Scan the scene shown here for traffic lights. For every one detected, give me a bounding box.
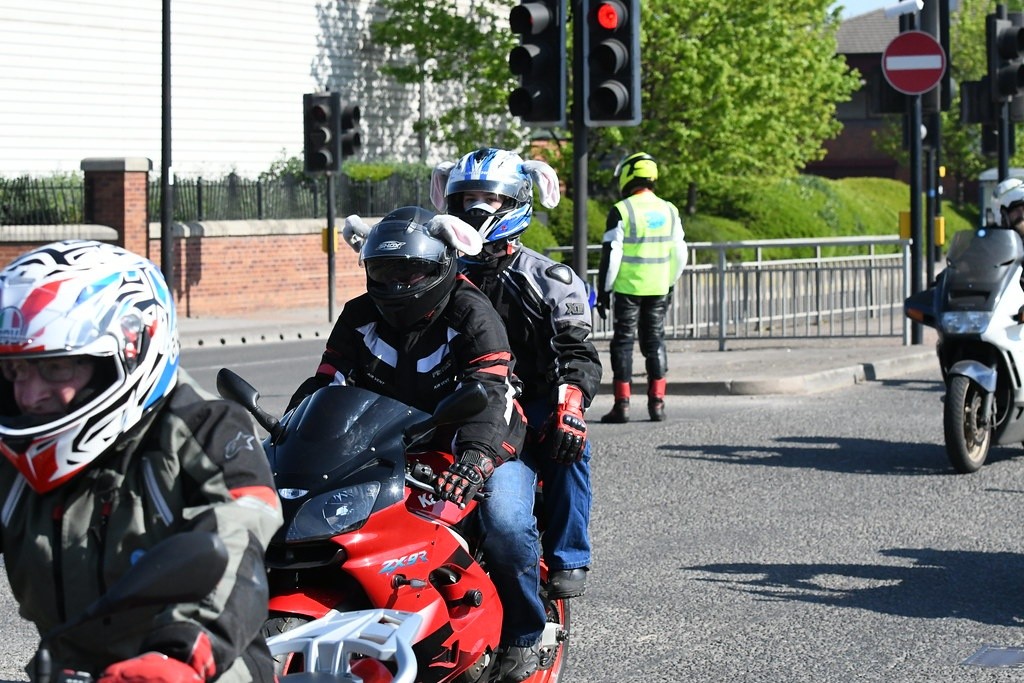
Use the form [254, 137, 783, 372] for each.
[507, 0, 568, 126]
[987, 14, 1024, 100]
[302, 91, 341, 172]
[940, 0, 960, 112]
[586, 1, 643, 129]
[341, 104, 363, 164]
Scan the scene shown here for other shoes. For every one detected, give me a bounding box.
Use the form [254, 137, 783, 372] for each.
[601, 403, 629, 423]
[548, 567, 586, 599]
[648, 399, 666, 420]
[499, 641, 540, 682]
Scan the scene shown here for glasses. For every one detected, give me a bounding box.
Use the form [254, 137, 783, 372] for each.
[0, 358, 104, 383]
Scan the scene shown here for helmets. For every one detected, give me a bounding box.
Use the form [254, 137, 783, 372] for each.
[444, 149, 533, 245]
[359, 206, 457, 329]
[613, 152, 658, 199]
[0, 239, 181, 499]
[990, 178, 1024, 227]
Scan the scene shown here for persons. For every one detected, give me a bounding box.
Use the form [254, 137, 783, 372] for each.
[0, 239, 307, 683]
[311, 207, 546, 682]
[936, 178, 1024, 292]
[596, 151, 688, 423]
[430, 148, 602, 599]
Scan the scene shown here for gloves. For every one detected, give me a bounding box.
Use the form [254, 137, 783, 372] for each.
[430, 449, 504, 510]
[668, 287, 674, 303]
[597, 291, 610, 319]
[536, 382, 590, 466]
[98, 622, 216, 683]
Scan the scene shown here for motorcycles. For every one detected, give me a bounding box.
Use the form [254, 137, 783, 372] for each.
[903, 222, 1024, 472]
[0, 366, 571, 683]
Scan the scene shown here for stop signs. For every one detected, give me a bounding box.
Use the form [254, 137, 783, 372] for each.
[883, 30, 945, 96]
